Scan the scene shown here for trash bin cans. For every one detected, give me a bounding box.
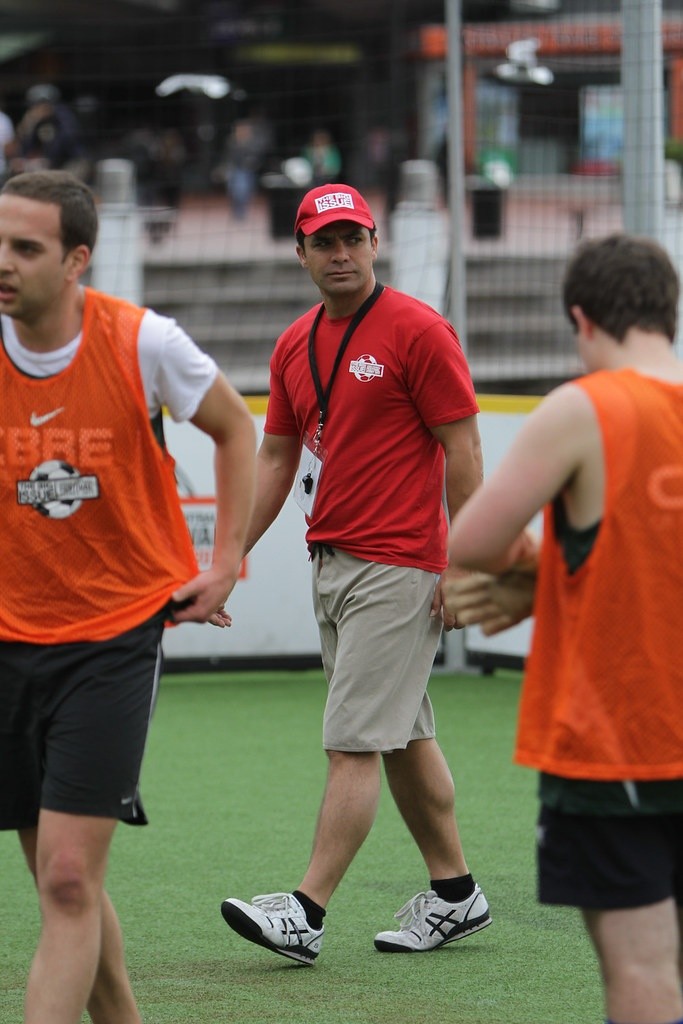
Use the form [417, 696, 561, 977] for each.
[471, 186, 504, 237]
[261, 187, 306, 242]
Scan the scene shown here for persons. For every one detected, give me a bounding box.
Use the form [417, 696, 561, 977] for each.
[0, 79, 399, 233]
[208, 184, 494, 965]
[441, 228, 683, 1023]
[0, 167, 259, 1024]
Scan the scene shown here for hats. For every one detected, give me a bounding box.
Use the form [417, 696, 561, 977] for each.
[294, 184, 374, 236]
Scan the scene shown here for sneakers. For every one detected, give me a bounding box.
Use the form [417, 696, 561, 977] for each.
[221, 892, 324, 966]
[373, 883, 492, 952]
